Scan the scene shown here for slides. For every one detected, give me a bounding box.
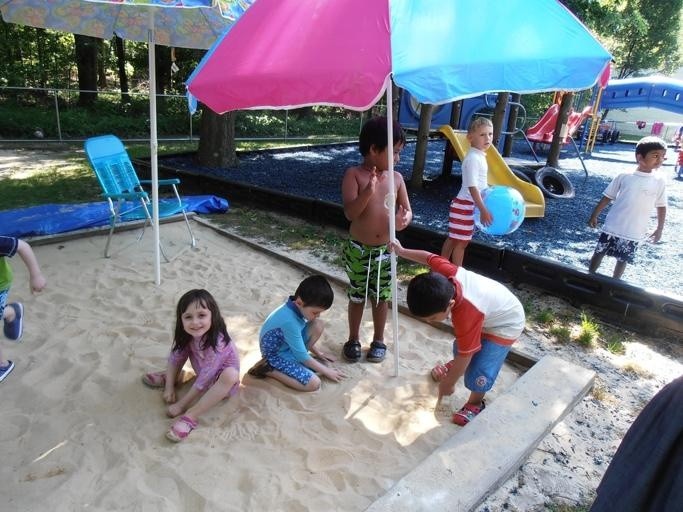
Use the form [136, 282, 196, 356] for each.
[529, 100, 591, 145]
[439, 123, 545, 218]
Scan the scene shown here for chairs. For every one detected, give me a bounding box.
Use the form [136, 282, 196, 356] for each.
[84, 134, 195, 263]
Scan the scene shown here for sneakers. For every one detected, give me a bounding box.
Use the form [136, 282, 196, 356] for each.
[142, 369, 178, 387]
[166, 414, 199, 443]
[248, 357, 275, 378]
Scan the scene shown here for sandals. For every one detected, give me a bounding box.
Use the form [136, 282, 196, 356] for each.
[343, 339, 362, 361]
[431, 360, 455, 383]
[453, 399, 485, 425]
[4, 301, 24, 342]
[0, 359, 15, 382]
[366, 341, 386, 362]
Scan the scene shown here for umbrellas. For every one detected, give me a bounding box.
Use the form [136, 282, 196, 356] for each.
[0, 0, 257, 284]
[186, 0, 616, 380]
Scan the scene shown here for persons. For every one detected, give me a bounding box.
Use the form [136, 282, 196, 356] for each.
[0, 235, 45, 382]
[588, 135, 668, 279]
[441, 118, 495, 269]
[247, 275, 347, 392]
[675, 147, 683, 180]
[386, 238, 526, 426]
[674, 127, 683, 153]
[342, 115, 412, 364]
[142, 289, 241, 443]
